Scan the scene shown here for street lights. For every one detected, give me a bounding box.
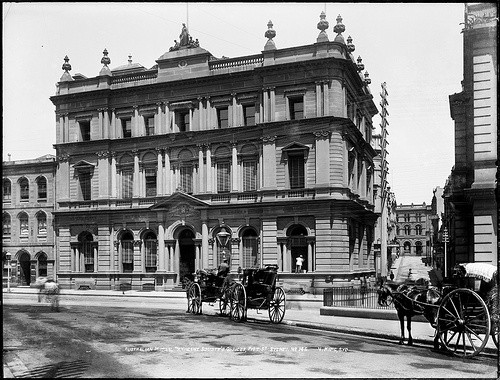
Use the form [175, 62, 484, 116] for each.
[5, 252, 12, 293]
[441, 224, 451, 275]
[217, 224, 232, 258]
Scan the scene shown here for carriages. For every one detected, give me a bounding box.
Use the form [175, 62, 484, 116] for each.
[375, 259, 499, 358]
[185, 258, 287, 325]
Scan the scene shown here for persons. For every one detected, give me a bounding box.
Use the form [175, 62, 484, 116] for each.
[217, 260, 229, 276]
[295, 255, 307, 273]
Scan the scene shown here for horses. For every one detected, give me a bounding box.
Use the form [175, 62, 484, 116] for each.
[376, 277, 448, 351]
